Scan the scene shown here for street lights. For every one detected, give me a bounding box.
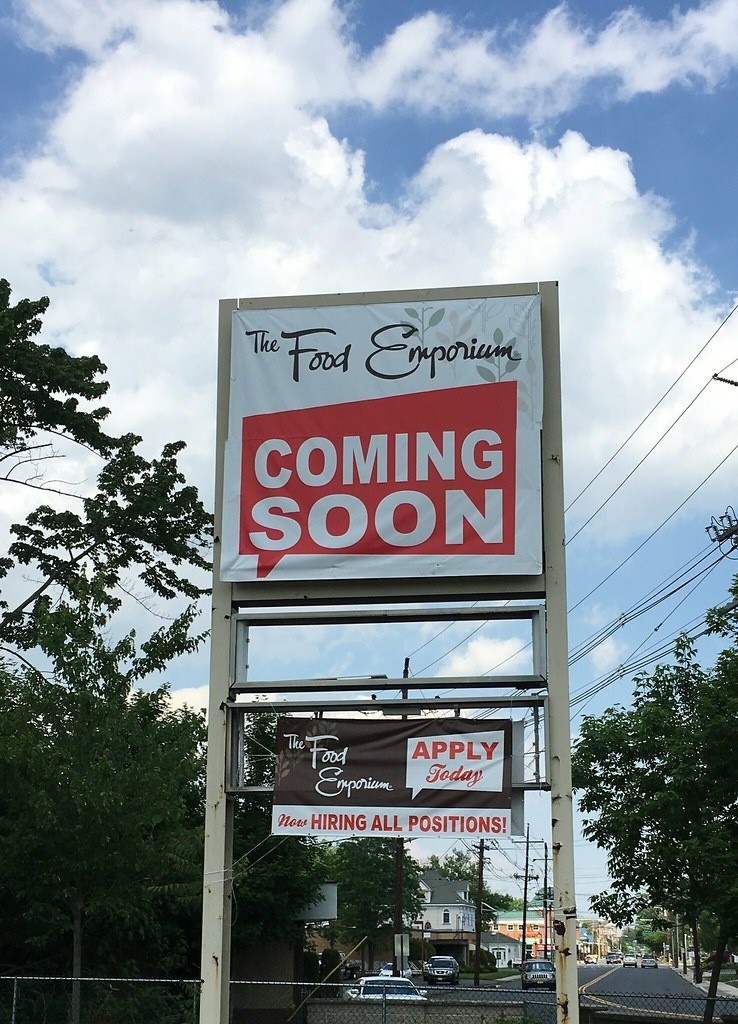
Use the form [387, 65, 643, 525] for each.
[414, 921, 424, 980]
[678, 925, 687, 974]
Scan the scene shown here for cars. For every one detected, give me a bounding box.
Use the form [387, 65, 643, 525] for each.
[423, 956, 453, 980]
[318, 951, 363, 980]
[379, 962, 412, 980]
[428, 958, 459, 985]
[636, 951, 642, 957]
[641, 955, 658, 968]
[606, 952, 624, 964]
[584, 955, 597, 964]
[519, 961, 556, 991]
[342, 976, 428, 1001]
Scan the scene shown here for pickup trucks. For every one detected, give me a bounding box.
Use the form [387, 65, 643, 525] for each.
[623, 954, 637, 968]
